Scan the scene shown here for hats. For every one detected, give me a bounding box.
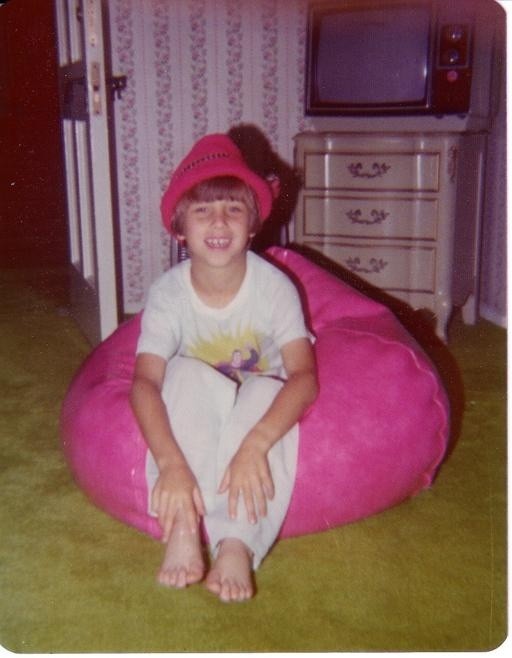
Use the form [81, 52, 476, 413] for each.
[162, 133, 273, 245]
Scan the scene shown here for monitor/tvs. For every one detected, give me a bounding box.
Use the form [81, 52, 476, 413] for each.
[302, 0, 477, 118]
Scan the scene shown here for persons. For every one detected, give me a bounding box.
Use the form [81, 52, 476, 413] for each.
[127, 134, 320, 601]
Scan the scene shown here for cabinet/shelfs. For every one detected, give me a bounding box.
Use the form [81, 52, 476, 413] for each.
[292, 133, 442, 345]
